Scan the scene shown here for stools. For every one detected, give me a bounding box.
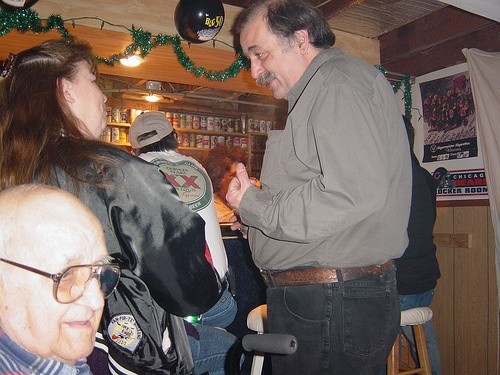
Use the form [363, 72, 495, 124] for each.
[386, 306, 433, 375]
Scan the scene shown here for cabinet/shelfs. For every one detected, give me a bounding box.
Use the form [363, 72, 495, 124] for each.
[99, 97, 279, 180]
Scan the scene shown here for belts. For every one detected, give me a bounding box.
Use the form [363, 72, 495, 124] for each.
[260, 259, 394, 287]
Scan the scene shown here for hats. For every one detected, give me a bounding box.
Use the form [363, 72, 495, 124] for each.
[129, 111, 173, 149]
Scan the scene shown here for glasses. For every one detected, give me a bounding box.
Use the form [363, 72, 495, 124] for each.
[0, 258, 121, 304]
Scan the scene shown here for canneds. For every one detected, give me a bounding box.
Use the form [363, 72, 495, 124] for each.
[99, 106, 280, 149]
[183, 315, 202, 326]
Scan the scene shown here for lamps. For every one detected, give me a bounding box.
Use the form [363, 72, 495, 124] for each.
[144, 81, 162, 103]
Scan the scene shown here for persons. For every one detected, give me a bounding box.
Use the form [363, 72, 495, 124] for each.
[225, 0, 412, 375]
[0, 183, 121, 375]
[205, 143, 263, 222]
[394, 114, 440, 375]
[0, 40, 226, 375]
[129, 112, 239, 329]
